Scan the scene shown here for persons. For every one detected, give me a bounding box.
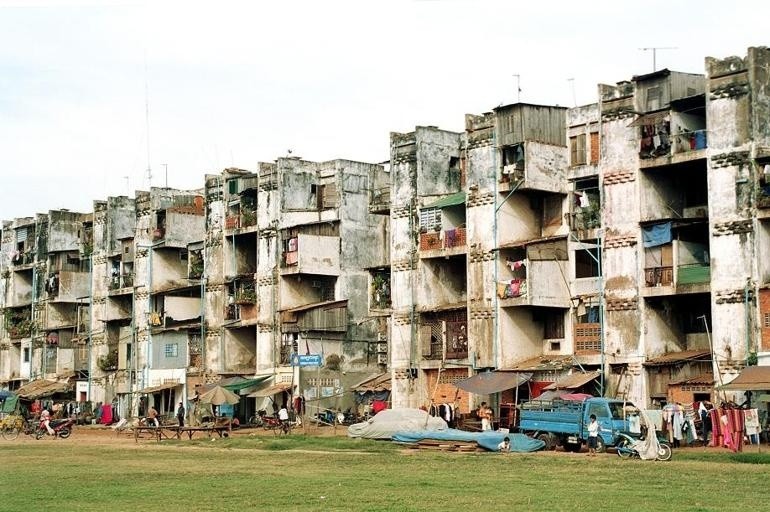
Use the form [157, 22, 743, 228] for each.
[277, 404, 290, 433]
[231, 414, 241, 429]
[496, 436, 511, 452]
[478, 401, 492, 432]
[584, 413, 598, 456]
[176, 401, 184, 426]
[35, 406, 55, 438]
[486, 406, 492, 420]
[215, 413, 232, 439]
[147, 406, 160, 428]
[139, 396, 144, 415]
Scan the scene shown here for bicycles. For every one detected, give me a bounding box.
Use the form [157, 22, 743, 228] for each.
[250, 410, 289, 434]
[3, 417, 31, 439]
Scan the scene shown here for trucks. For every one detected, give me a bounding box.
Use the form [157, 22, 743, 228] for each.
[519, 396, 642, 453]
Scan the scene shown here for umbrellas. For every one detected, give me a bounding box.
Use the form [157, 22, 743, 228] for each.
[197, 384, 241, 418]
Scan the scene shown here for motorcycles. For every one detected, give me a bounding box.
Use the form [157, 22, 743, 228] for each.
[615, 423, 672, 460]
[36, 418, 76, 440]
